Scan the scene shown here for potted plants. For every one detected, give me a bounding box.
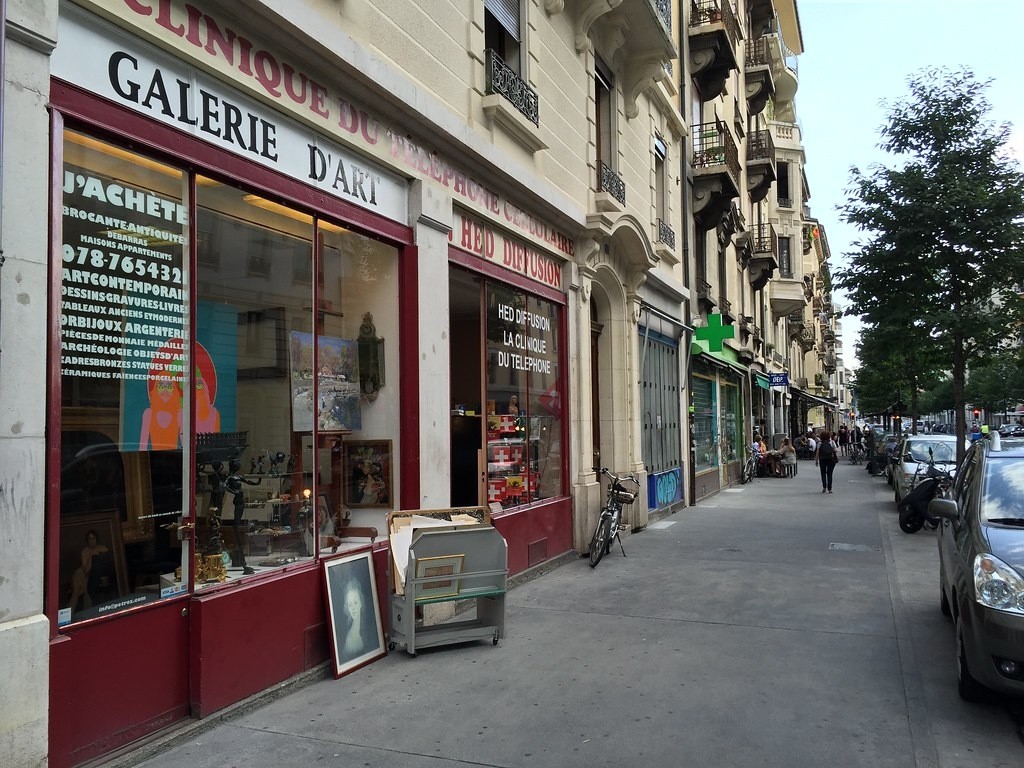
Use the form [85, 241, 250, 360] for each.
[707, 6, 722, 24]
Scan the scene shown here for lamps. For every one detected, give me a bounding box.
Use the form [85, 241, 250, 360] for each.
[242, 194, 351, 234]
[63, 128, 222, 189]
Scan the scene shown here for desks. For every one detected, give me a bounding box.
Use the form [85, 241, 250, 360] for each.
[765, 454, 782, 478]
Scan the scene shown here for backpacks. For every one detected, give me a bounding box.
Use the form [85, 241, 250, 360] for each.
[819, 440, 832, 456]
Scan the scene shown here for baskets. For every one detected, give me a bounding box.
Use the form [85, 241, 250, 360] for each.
[608, 484, 638, 505]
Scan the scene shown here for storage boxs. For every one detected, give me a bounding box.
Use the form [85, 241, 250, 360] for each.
[488, 416, 541, 507]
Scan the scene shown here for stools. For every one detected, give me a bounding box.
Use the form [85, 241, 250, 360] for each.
[781, 462, 798, 478]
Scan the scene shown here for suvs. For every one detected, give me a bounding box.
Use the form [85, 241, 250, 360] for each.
[927, 427, 1024, 707]
[57, 441, 187, 577]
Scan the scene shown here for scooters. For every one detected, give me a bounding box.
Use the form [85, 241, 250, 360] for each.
[898, 446, 957, 535]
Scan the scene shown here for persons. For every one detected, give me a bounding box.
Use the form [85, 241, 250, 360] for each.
[752, 435, 796, 478]
[793, 431, 820, 460]
[223, 460, 262, 547]
[923, 423, 934, 435]
[969, 419, 989, 437]
[816, 431, 838, 494]
[1014, 418, 1024, 426]
[508, 396, 518, 413]
[836, 425, 863, 456]
[832, 433, 837, 443]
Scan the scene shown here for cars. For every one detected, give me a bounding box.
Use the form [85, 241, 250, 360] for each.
[891, 436, 971, 505]
[997, 423, 1024, 438]
[872, 423, 912, 455]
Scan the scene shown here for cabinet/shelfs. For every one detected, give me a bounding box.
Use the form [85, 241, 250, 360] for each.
[389, 517, 510, 658]
[193, 471, 327, 557]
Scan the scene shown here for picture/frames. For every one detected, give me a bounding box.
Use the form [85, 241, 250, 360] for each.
[59, 508, 130, 616]
[60, 406, 155, 545]
[247, 226, 272, 281]
[343, 440, 393, 510]
[414, 554, 466, 601]
[238, 307, 288, 380]
[320, 544, 387, 679]
[292, 241, 324, 288]
[196, 211, 222, 270]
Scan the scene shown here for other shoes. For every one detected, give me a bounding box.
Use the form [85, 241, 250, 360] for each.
[829, 490, 833, 493]
[822, 489, 826, 492]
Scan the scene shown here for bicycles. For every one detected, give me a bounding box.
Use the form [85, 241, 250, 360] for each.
[901, 451, 926, 495]
[847, 442, 864, 466]
[588, 467, 640, 568]
[740, 444, 761, 484]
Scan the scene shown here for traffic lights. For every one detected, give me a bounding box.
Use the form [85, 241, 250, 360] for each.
[975, 410, 979, 420]
